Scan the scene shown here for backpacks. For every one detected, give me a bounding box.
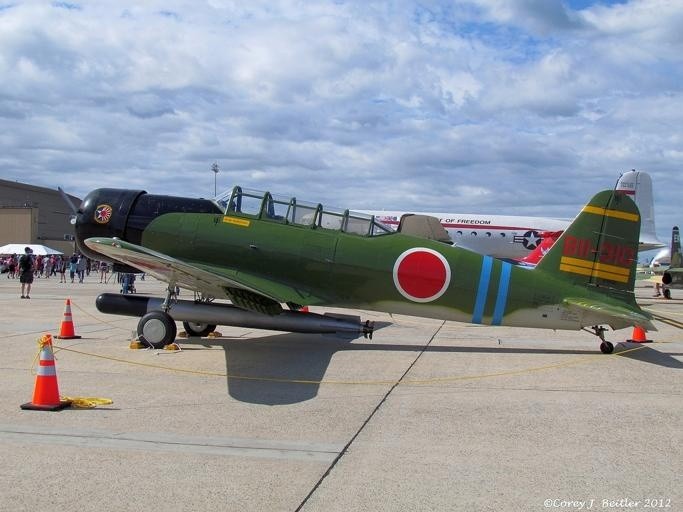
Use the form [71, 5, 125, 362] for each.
[20, 255, 33, 271]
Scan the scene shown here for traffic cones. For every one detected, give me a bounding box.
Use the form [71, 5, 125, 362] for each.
[626, 326, 653, 343]
[55, 298, 82, 339]
[21, 334, 73, 409]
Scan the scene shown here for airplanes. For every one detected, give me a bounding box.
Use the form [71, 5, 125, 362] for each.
[302, 171, 662, 262]
[650, 225, 683, 275]
[59, 189, 658, 353]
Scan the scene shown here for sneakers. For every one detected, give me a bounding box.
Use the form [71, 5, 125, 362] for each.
[21, 295, 30, 298]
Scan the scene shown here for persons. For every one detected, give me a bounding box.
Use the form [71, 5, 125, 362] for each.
[0, 246, 146, 299]
[652, 261, 664, 297]
